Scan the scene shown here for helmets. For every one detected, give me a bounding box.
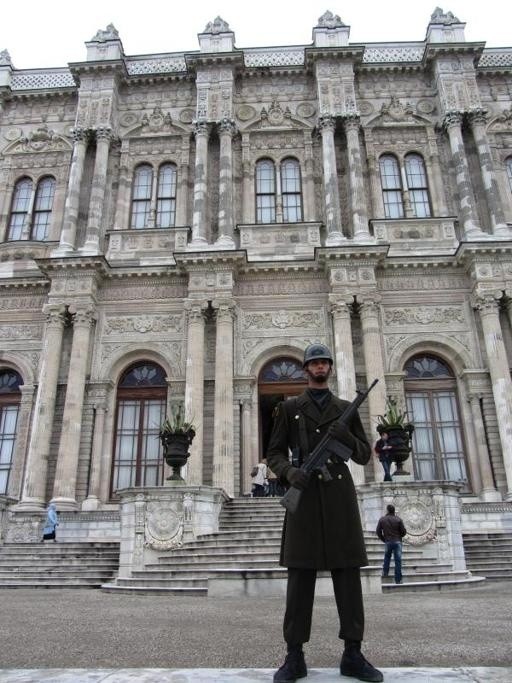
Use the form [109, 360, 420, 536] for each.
[302, 343, 334, 368]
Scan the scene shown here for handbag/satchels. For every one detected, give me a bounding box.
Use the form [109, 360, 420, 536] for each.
[250, 466, 258, 477]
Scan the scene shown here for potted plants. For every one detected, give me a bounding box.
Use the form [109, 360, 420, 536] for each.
[371, 393, 419, 475]
[151, 401, 203, 480]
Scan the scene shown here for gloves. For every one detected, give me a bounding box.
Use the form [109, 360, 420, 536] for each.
[282, 465, 310, 491]
[327, 419, 355, 449]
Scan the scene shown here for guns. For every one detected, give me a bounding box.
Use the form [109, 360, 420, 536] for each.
[280, 378, 378, 515]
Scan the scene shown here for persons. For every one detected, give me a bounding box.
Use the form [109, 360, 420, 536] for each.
[251, 458, 268, 497]
[268, 467, 279, 497]
[376, 505, 406, 584]
[41, 504, 59, 543]
[268, 345, 383, 683]
[375, 433, 393, 481]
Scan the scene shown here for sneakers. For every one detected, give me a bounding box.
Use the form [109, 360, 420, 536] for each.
[272, 650, 308, 682]
[395, 576, 403, 584]
[338, 648, 385, 682]
[382, 573, 389, 577]
